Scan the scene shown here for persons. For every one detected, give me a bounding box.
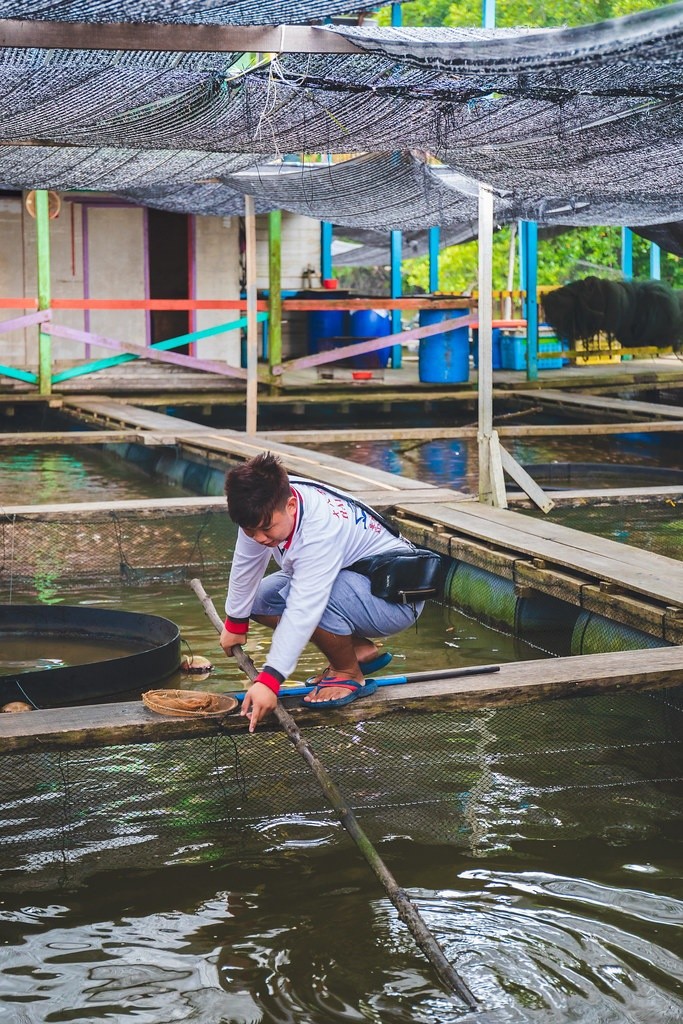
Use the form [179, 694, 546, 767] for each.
[219, 449, 428, 735]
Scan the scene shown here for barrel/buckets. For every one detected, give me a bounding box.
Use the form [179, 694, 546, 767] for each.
[347, 295, 391, 366]
[419, 295, 471, 384]
[309, 290, 350, 364]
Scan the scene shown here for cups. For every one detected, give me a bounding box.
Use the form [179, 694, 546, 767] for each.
[323, 279, 338, 289]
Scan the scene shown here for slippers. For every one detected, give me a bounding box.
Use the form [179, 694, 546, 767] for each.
[299, 653, 393, 708]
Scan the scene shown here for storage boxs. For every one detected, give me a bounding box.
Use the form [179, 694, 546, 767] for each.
[472, 319, 623, 370]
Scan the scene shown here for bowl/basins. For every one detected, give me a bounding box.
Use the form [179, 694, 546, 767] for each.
[353, 372, 373, 378]
[321, 374, 334, 379]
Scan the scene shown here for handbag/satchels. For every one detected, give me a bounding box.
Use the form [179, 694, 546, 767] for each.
[352, 548, 442, 603]
